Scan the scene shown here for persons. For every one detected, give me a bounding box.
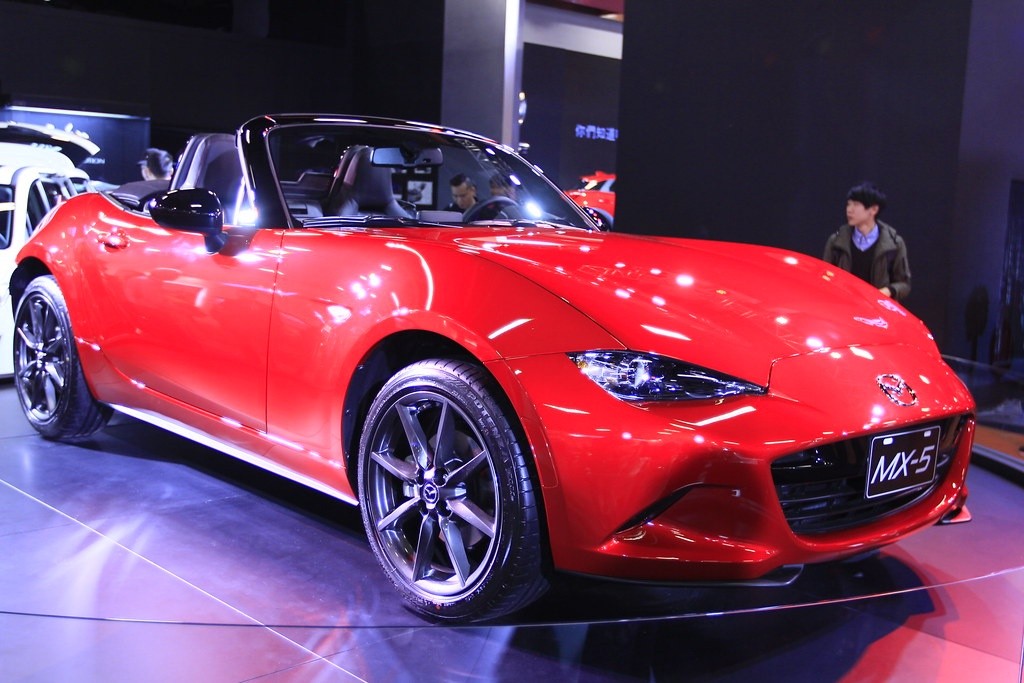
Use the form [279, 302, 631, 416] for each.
[823, 183, 911, 299]
[443, 175, 479, 213]
[489, 174, 515, 203]
[141, 149, 174, 180]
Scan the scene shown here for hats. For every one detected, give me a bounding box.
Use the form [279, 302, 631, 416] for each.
[137, 148, 159, 165]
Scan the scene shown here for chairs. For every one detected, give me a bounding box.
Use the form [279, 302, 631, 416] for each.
[170, 131, 261, 227]
[325, 147, 416, 223]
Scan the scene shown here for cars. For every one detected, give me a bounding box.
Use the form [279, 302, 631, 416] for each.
[562, 170, 617, 232]
[0, 121, 101, 381]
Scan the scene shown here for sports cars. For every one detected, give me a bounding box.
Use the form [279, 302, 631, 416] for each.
[5, 112, 977, 626]
